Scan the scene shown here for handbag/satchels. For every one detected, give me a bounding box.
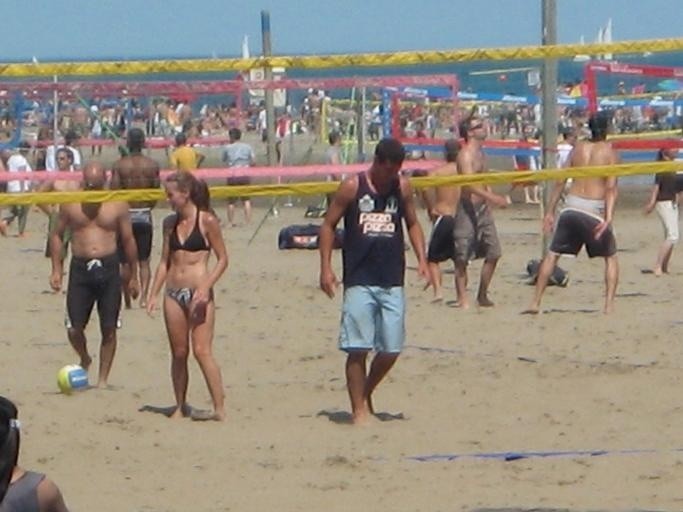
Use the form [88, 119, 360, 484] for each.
[304, 204, 330, 218]
[277, 219, 349, 252]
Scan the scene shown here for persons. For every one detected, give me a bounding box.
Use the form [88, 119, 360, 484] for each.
[318, 138, 432, 423]
[0, 83, 683, 234]
[47, 160, 141, 390]
[643, 140, 682, 278]
[520, 113, 619, 317]
[142, 174, 228, 423]
[450, 112, 510, 308]
[109, 129, 159, 309]
[423, 137, 466, 303]
[0, 393, 70, 510]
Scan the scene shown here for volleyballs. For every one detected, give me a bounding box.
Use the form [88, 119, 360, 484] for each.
[57, 364, 88, 396]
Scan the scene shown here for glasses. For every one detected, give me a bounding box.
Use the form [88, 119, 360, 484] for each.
[469, 122, 484, 130]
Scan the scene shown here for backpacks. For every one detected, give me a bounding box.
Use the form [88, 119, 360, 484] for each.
[523, 256, 572, 288]
